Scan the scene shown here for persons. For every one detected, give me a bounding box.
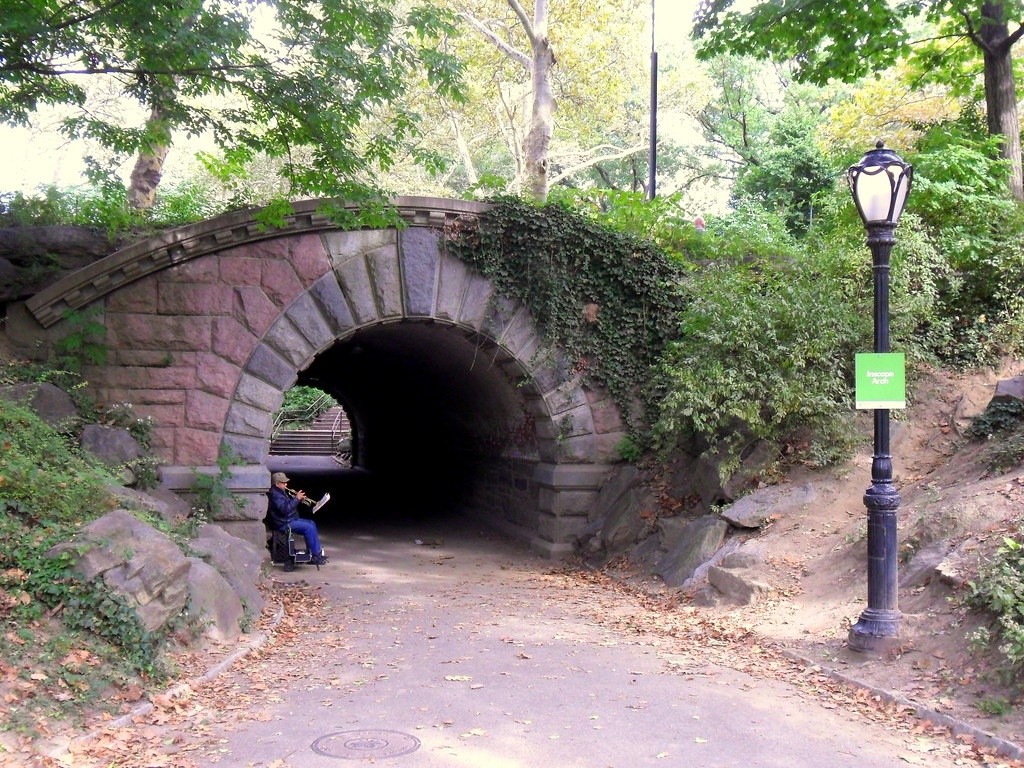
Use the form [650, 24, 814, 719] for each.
[268, 472, 326, 565]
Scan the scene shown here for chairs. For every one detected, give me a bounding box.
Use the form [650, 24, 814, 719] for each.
[266, 492, 315, 565]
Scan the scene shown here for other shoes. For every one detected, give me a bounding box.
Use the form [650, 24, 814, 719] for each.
[311, 554, 327, 564]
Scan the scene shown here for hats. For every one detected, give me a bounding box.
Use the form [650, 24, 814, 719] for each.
[272, 472, 290, 483]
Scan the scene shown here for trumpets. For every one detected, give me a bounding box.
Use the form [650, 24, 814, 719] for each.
[285, 486, 322, 507]
